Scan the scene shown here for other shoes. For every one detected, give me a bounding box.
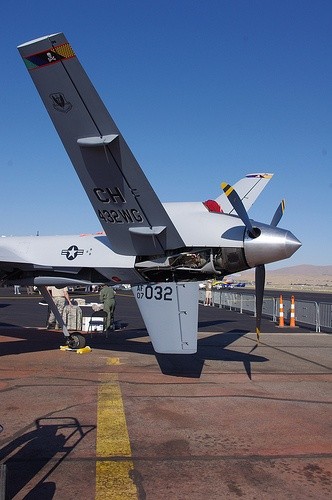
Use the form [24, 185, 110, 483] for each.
[55, 325, 62, 330]
[46, 324, 54, 329]
[104, 328, 108, 332]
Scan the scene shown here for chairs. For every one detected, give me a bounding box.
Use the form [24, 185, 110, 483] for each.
[86, 310, 109, 341]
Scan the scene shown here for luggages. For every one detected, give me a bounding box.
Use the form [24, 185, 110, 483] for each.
[62, 305, 83, 331]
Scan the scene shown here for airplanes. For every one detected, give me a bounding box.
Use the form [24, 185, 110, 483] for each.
[0, 31, 301, 354]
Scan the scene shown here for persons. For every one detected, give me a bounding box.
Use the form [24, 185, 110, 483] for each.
[99, 285, 117, 332]
[46, 286, 73, 330]
[205, 280, 212, 305]
[14, 285, 20, 295]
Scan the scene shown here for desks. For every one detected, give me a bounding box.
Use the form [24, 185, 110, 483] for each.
[79, 304, 107, 332]
